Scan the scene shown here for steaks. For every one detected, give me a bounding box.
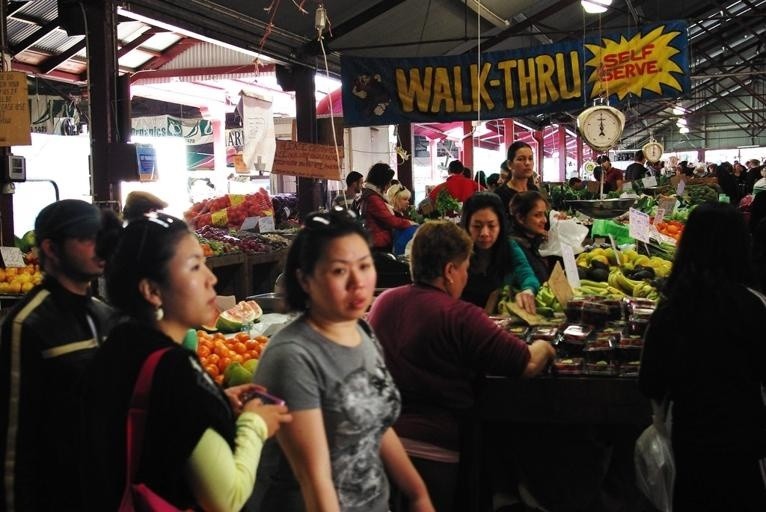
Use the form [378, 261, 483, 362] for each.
[576, 247, 673, 279]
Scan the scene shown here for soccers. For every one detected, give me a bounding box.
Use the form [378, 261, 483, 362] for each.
[184, 188, 274, 227]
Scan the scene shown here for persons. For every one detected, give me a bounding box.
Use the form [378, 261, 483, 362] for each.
[637, 200, 765, 512]
[90, 209, 292, 512]
[332, 143, 562, 316]
[370, 219, 557, 510]
[251, 224, 437, 511]
[569, 149, 766, 213]
[1, 200, 117, 512]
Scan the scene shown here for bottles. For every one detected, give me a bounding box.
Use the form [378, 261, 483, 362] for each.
[126, 191, 167, 210]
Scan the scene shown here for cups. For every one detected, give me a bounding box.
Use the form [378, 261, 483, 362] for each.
[136, 212, 175, 263]
[303, 208, 357, 232]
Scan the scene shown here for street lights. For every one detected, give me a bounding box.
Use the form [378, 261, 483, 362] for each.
[119, 483, 181, 512]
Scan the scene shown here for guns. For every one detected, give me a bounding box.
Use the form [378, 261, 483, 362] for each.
[497, 265, 660, 315]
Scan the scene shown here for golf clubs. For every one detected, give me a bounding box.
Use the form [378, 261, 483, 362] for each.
[201, 299, 264, 333]
[12, 230, 36, 252]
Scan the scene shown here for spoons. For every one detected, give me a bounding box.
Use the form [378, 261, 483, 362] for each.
[650, 216, 686, 241]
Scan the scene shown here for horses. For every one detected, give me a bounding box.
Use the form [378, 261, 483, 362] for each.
[238, 384, 287, 427]
